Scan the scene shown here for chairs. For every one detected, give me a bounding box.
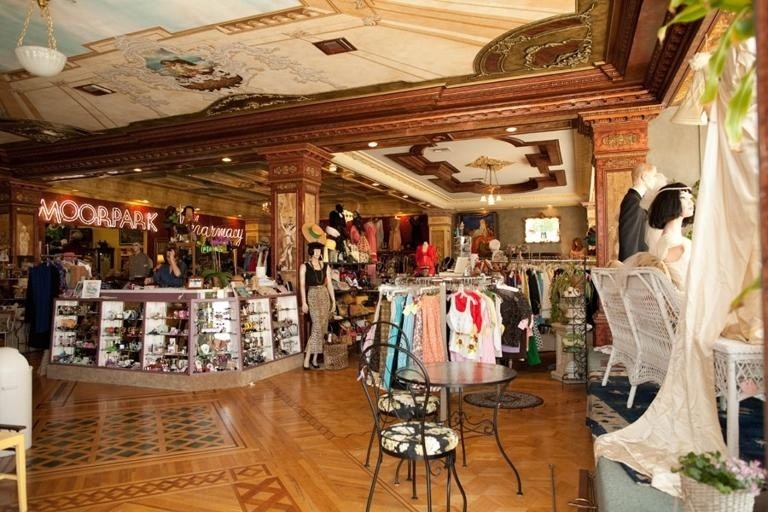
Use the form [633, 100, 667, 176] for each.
[593, 268, 640, 409]
[358, 344, 467, 512]
[627, 266, 695, 411]
[357, 322, 447, 483]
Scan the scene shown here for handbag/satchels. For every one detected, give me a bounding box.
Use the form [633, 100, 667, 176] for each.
[325, 324, 337, 344]
[359, 252, 369, 264]
[343, 294, 353, 304]
[357, 235, 370, 253]
[349, 305, 364, 316]
[339, 329, 352, 346]
[355, 316, 369, 333]
[336, 304, 350, 316]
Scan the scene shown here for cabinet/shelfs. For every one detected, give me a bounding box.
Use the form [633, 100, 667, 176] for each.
[329, 263, 380, 345]
[50, 295, 303, 374]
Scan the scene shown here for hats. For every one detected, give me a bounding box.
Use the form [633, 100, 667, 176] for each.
[331, 269, 340, 282]
[339, 320, 352, 332]
[326, 239, 336, 251]
[302, 223, 326, 245]
[351, 226, 361, 244]
[445, 257, 454, 268]
[332, 278, 350, 291]
[308, 242, 325, 248]
[326, 226, 340, 238]
[355, 208, 360, 216]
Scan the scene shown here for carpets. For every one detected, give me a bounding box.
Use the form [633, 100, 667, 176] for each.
[464, 390, 545, 409]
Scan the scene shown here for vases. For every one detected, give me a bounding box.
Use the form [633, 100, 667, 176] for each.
[681, 479, 759, 512]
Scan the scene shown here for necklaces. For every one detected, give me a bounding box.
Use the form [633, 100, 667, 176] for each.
[310, 260, 324, 285]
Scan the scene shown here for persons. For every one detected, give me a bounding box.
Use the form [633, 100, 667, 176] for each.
[329, 204, 348, 261]
[618, 163, 657, 262]
[144, 248, 187, 288]
[569, 238, 588, 259]
[127, 240, 150, 284]
[648, 182, 694, 290]
[299, 243, 337, 371]
[416, 242, 437, 277]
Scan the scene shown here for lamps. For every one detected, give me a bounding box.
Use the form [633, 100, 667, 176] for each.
[15, 3, 66, 77]
[476, 165, 504, 205]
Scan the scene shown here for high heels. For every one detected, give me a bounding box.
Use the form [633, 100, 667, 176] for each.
[303, 363, 310, 370]
[312, 361, 320, 368]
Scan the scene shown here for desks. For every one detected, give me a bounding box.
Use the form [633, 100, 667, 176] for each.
[712, 336, 767, 463]
[394, 360, 522, 511]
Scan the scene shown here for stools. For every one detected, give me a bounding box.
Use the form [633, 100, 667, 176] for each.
[0, 430, 28, 512]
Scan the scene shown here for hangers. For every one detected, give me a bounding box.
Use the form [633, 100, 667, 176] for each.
[40, 253, 83, 264]
[474, 259, 596, 270]
[377, 276, 501, 298]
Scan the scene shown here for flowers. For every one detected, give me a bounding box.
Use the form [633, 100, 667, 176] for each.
[673, 448, 768, 496]
